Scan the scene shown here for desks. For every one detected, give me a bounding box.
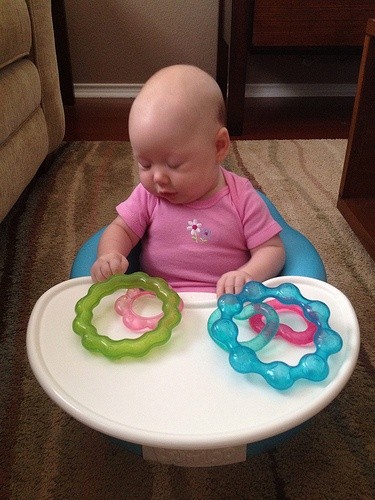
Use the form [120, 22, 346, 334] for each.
[26, 275, 360, 451]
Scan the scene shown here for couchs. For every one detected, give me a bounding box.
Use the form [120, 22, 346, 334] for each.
[0, 0, 67, 229]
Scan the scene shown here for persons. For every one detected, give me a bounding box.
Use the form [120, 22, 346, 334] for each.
[89, 64, 287, 299]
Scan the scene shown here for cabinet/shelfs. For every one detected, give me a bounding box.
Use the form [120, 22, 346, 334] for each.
[218, 0, 375, 259]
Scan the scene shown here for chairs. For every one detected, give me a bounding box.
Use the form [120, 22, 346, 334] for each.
[70, 191, 327, 281]
